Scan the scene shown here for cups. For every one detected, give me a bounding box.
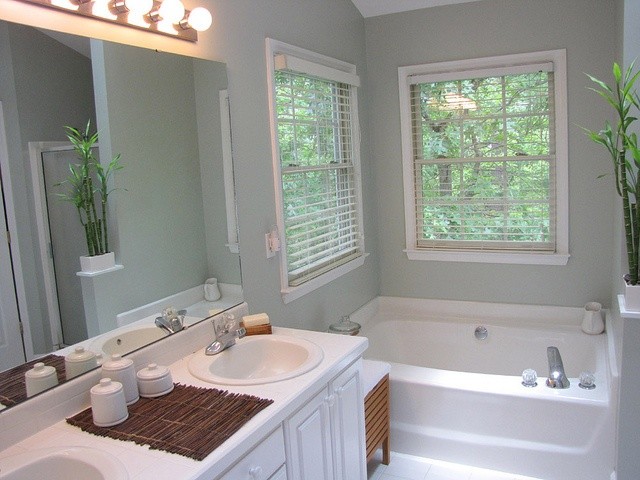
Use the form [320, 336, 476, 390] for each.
[582, 302, 604, 334]
[204, 277, 221, 301]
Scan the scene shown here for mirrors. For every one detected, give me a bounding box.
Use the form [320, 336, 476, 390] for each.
[0, 18, 247, 412]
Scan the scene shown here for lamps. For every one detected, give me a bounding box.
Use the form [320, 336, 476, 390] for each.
[92, 0, 117, 21]
[149, 0, 185, 25]
[51, 0, 80, 11]
[178, 7, 213, 32]
[127, 12, 153, 28]
[116, 0, 153, 14]
[157, 19, 182, 35]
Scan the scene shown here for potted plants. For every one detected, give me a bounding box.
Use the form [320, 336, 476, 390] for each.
[53, 117, 128, 272]
[574, 56, 640, 312]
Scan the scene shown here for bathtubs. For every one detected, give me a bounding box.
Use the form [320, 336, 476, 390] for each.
[361, 320, 597, 378]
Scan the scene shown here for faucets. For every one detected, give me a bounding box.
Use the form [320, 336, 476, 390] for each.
[205, 313, 246, 355]
[545, 346, 570, 389]
[155, 306, 187, 334]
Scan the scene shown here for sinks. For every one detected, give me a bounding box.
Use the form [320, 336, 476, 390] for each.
[89, 326, 169, 356]
[186, 335, 323, 384]
[0, 454, 105, 480]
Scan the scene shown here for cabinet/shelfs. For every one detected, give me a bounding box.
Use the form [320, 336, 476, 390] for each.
[282, 348, 368, 480]
[194, 417, 287, 480]
